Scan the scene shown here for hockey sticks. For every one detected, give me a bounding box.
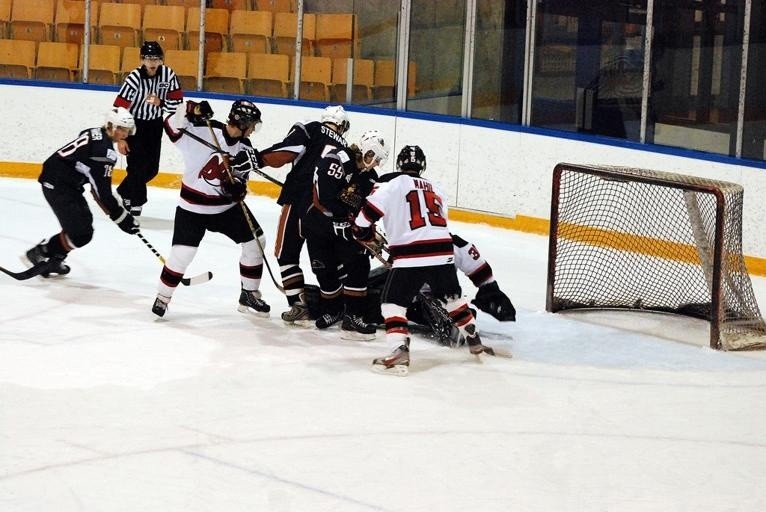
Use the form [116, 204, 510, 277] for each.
[136, 230, 213, 287]
[204, 121, 288, 296]
[375, 244, 518, 361]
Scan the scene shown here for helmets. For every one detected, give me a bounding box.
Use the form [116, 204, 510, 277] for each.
[355, 131, 389, 170]
[229, 100, 262, 132]
[140, 41, 163, 66]
[105, 107, 136, 138]
[319, 104, 350, 132]
[396, 146, 425, 173]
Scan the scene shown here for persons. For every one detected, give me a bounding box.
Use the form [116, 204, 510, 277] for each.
[229, 103, 518, 368]
[26, 106, 141, 279]
[113, 40, 183, 216]
[152, 99, 271, 317]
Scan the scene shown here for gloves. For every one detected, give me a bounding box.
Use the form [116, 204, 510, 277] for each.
[225, 176, 246, 195]
[229, 147, 264, 175]
[110, 207, 139, 234]
[194, 101, 213, 122]
[333, 216, 373, 242]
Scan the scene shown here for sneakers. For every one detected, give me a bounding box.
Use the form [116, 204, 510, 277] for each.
[152, 298, 167, 317]
[27, 245, 70, 277]
[374, 345, 409, 366]
[281, 301, 386, 333]
[123, 197, 142, 215]
[239, 290, 270, 312]
[467, 332, 495, 354]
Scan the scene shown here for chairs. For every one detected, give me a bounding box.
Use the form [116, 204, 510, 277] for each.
[1, 1, 415, 108]
